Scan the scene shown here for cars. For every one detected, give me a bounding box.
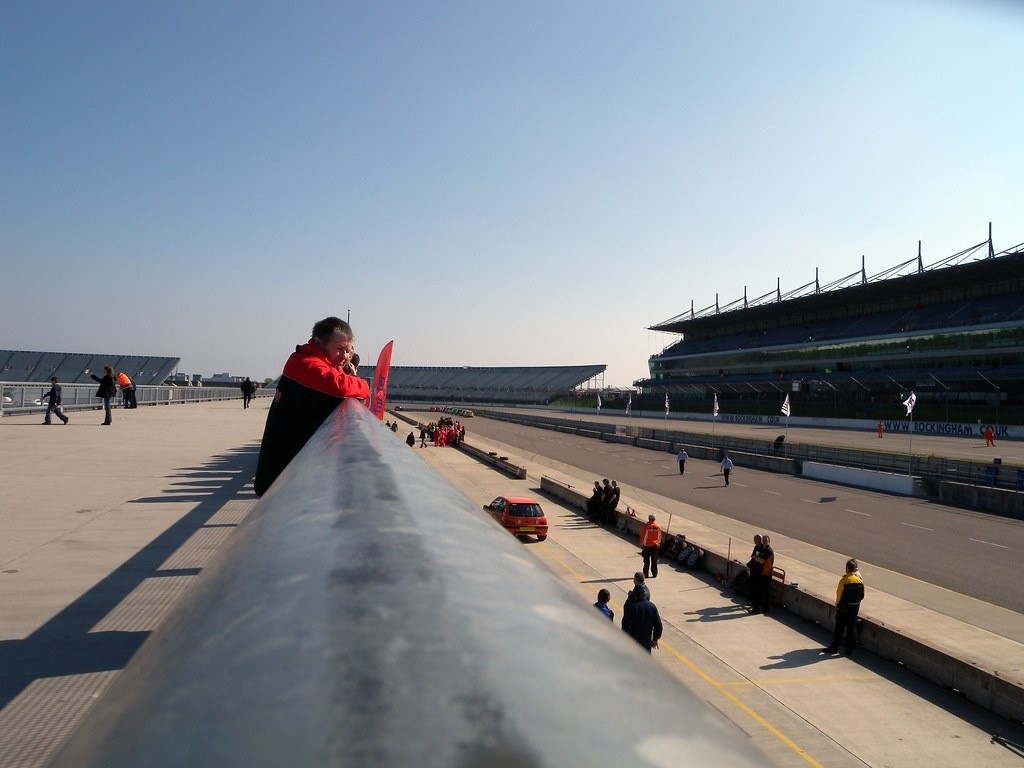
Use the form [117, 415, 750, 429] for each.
[428, 406, 474, 417]
[483, 496, 548, 541]
[395, 406, 401, 410]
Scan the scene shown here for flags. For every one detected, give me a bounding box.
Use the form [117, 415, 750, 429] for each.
[780, 394, 790, 417]
[713, 395, 719, 416]
[902, 393, 916, 416]
[596, 395, 602, 411]
[626, 396, 632, 414]
[665, 395, 670, 415]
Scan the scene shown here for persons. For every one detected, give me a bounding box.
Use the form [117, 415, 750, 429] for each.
[40, 377, 70, 425]
[241, 376, 257, 409]
[188, 379, 194, 386]
[392, 421, 399, 432]
[117, 372, 138, 408]
[822, 556, 865, 655]
[197, 380, 202, 387]
[406, 432, 415, 447]
[675, 448, 688, 475]
[586, 478, 620, 528]
[419, 429, 427, 447]
[984, 426, 995, 447]
[877, 420, 884, 438]
[593, 570, 663, 656]
[720, 454, 733, 487]
[426, 413, 465, 447]
[89, 365, 117, 425]
[744, 534, 774, 617]
[640, 513, 662, 578]
[386, 420, 390, 427]
[253, 317, 370, 499]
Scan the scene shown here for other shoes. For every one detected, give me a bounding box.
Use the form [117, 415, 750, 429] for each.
[42, 421, 51, 424]
[64, 417, 68, 425]
[822, 648, 838, 653]
[101, 422, 110, 425]
